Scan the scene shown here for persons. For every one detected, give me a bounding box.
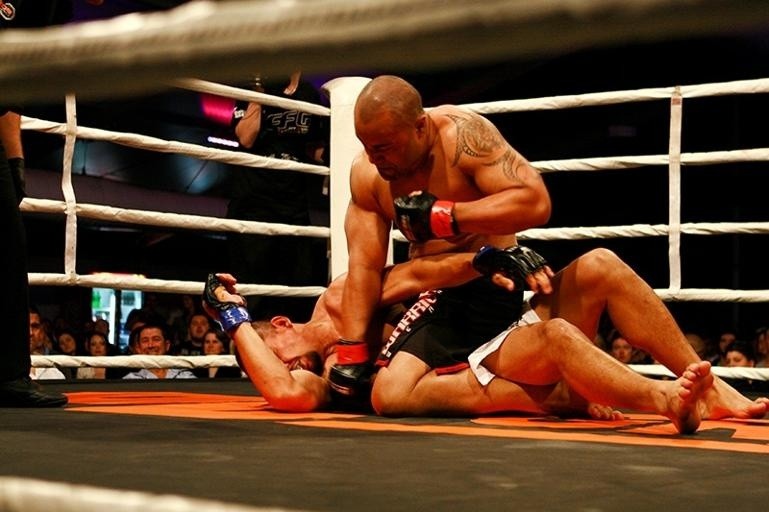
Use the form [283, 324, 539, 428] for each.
[28, 294, 247, 380]
[0, 0, 70, 410]
[226, 64, 328, 321]
[200, 244, 768, 433]
[326, 74, 633, 424]
[594, 329, 672, 381]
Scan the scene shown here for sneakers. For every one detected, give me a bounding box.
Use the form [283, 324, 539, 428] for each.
[1, 378, 68, 407]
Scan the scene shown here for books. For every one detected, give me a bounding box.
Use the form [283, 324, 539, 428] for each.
[684, 329, 769, 401]
